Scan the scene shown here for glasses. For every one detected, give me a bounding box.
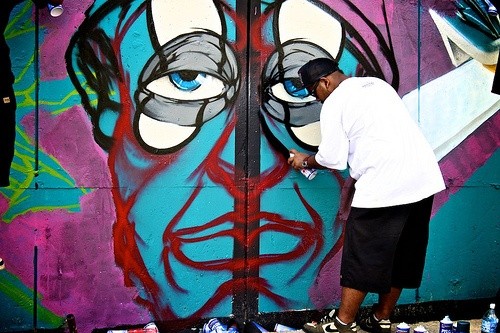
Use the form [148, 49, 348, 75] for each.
[311, 81, 320, 97]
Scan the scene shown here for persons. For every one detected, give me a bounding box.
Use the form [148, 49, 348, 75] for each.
[288, 59, 447, 333]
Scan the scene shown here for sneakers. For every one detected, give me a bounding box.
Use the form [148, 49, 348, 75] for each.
[304, 308, 357, 333]
[360, 314, 392, 333]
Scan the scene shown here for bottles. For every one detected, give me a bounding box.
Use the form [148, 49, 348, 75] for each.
[481, 304, 498, 333]
[414, 325, 427, 333]
[439, 316, 454, 333]
[396, 322, 411, 333]
[290, 153, 317, 181]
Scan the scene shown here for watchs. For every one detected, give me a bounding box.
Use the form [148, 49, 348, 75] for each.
[302, 155, 313, 167]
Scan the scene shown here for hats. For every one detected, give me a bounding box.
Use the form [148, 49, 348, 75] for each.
[291, 58, 339, 92]
